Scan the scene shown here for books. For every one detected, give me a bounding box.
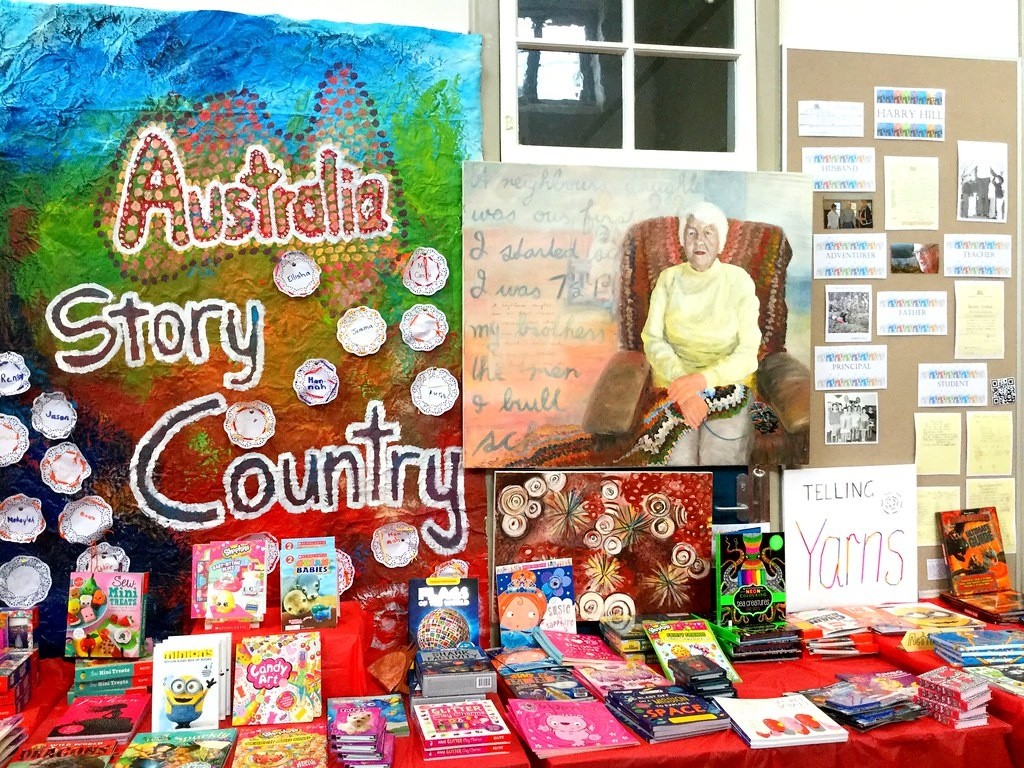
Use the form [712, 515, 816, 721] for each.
[2, 505, 1024, 766]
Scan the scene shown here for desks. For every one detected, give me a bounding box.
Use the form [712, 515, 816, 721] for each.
[6, 660, 532, 768]
[874, 623, 1023, 732]
[498, 628, 1016, 768]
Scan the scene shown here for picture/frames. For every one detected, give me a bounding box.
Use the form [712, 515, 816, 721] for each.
[2, 0, 483, 646]
[458, 157, 814, 468]
[492, 472, 713, 625]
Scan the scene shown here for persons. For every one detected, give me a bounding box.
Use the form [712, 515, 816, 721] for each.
[826, 203, 839, 229]
[914, 244, 938, 273]
[825, 393, 875, 443]
[854, 200, 872, 227]
[839, 201, 856, 229]
